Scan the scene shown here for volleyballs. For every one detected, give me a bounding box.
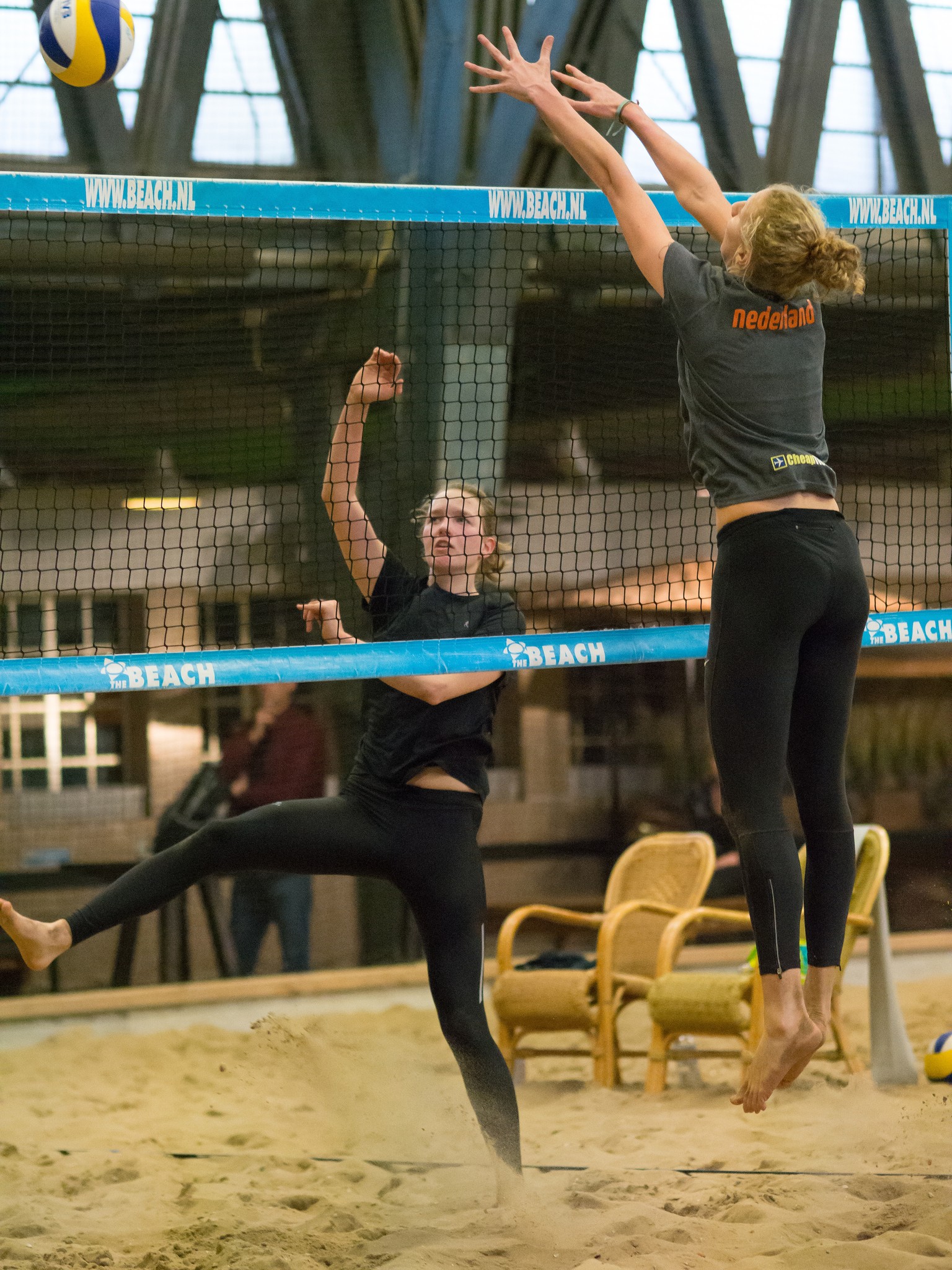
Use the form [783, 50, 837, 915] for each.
[923, 1030, 952, 1085]
[38, 0, 135, 88]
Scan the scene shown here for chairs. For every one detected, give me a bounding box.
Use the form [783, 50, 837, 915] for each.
[492, 831, 716, 1086]
[647, 824, 890, 1094]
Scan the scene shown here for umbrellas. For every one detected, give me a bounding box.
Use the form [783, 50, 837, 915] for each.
[564, 549, 922, 802]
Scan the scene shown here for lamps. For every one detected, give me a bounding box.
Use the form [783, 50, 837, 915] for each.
[121, 448, 202, 511]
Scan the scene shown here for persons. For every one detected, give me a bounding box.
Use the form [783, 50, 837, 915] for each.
[224, 677, 334, 975]
[2, 342, 529, 1180]
[464, 25, 866, 1114]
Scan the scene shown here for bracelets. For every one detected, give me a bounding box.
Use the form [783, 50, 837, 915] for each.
[605, 98, 635, 136]
